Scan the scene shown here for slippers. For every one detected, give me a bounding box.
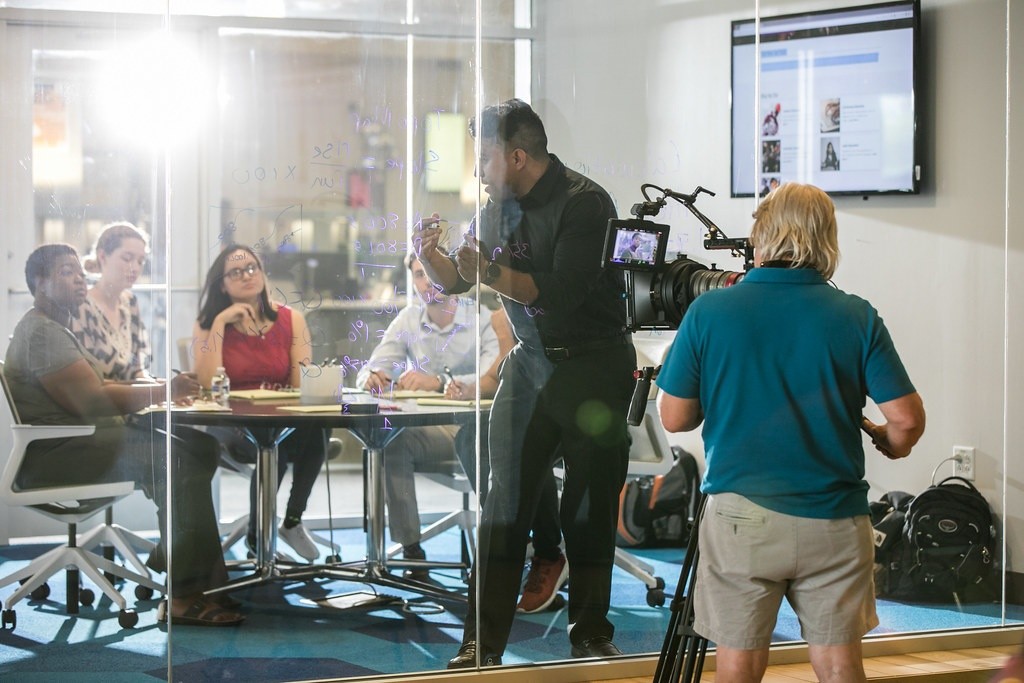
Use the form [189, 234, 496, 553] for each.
[213, 593, 242, 608]
[164, 594, 246, 627]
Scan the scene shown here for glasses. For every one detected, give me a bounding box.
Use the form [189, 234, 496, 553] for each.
[221, 262, 259, 281]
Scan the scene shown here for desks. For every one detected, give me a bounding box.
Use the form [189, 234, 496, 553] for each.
[136, 395, 491, 622]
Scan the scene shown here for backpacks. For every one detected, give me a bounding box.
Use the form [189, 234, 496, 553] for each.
[897, 476, 997, 604]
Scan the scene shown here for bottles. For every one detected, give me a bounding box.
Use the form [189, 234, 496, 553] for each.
[212, 367, 230, 406]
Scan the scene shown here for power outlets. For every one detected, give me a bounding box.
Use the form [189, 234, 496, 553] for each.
[953, 446, 975, 481]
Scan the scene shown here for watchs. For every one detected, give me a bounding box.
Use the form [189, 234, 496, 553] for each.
[482, 262, 502, 287]
[437, 373, 446, 393]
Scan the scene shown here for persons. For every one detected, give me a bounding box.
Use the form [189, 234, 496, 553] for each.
[621, 233, 643, 260]
[763, 102, 780, 136]
[410, 98, 638, 669]
[822, 143, 839, 170]
[762, 142, 780, 173]
[656, 184, 925, 683]
[191, 244, 333, 560]
[355, 245, 500, 582]
[0, 244, 246, 626]
[57, 224, 221, 575]
[761, 178, 779, 196]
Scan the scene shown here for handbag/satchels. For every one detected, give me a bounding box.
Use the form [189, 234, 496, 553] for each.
[615, 445, 702, 546]
[868, 489, 915, 601]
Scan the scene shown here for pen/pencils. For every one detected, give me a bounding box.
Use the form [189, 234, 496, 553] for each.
[380, 405, 401, 411]
[171, 367, 206, 391]
[299, 357, 339, 366]
[369, 369, 400, 383]
[444, 366, 455, 381]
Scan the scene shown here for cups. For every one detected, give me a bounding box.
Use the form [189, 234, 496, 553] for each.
[300, 366, 343, 404]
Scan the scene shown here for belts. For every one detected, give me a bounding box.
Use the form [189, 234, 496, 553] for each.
[523, 333, 632, 362]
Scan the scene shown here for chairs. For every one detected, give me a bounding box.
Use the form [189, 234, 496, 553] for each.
[0, 361, 483, 629]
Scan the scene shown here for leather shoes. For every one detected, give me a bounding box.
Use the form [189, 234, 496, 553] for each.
[447, 640, 502, 668]
[571, 636, 623, 658]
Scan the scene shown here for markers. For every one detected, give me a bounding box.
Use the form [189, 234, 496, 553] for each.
[428, 223, 441, 228]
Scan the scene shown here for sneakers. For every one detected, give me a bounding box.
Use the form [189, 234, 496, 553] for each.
[245, 536, 296, 562]
[278, 521, 320, 558]
[514, 550, 570, 613]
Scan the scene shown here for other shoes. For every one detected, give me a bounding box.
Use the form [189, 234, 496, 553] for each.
[402, 543, 427, 581]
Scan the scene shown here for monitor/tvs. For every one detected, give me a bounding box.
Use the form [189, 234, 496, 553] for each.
[728, 0, 922, 197]
